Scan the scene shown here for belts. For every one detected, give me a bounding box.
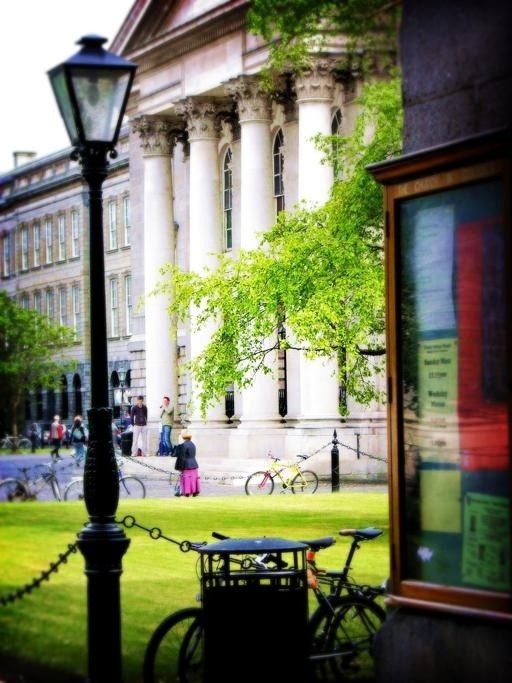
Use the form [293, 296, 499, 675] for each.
[135, 423, 145, 425]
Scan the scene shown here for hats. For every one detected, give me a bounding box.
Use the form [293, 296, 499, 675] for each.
[182, 433, 192, 439]
[53, 415, 61, 420]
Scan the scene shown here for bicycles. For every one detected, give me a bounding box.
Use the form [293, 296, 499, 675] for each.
[0, 434, 32, 450]
[243, 453, 322, 493]
[142, 526, 391, 683]
[3, 455, 151, 505]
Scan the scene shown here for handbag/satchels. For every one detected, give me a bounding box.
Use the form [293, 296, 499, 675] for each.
[72, 429, 83, 440]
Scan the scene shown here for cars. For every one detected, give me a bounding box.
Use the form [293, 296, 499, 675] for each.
[22, 419, 122, 449]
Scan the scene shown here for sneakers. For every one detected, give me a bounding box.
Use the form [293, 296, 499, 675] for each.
[162, 451, 172, 455]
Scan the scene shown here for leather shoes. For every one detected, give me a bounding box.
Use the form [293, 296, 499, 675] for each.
[185, 492, 198, 496]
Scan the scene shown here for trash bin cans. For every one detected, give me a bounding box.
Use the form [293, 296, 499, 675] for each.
[199, 539, 310, 683]
[113, 406, 119, 418]
[121, 432, 133, 456]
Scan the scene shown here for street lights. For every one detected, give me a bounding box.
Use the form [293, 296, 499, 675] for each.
[115, 360, 134, 455]
[49, 32, 136, 683]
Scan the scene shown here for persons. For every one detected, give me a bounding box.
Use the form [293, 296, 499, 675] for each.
[112, 395, 151, 457]
[172, 431, 201, 497]
[28, 413, 88, 466]
[159, 396, 173, 455]
